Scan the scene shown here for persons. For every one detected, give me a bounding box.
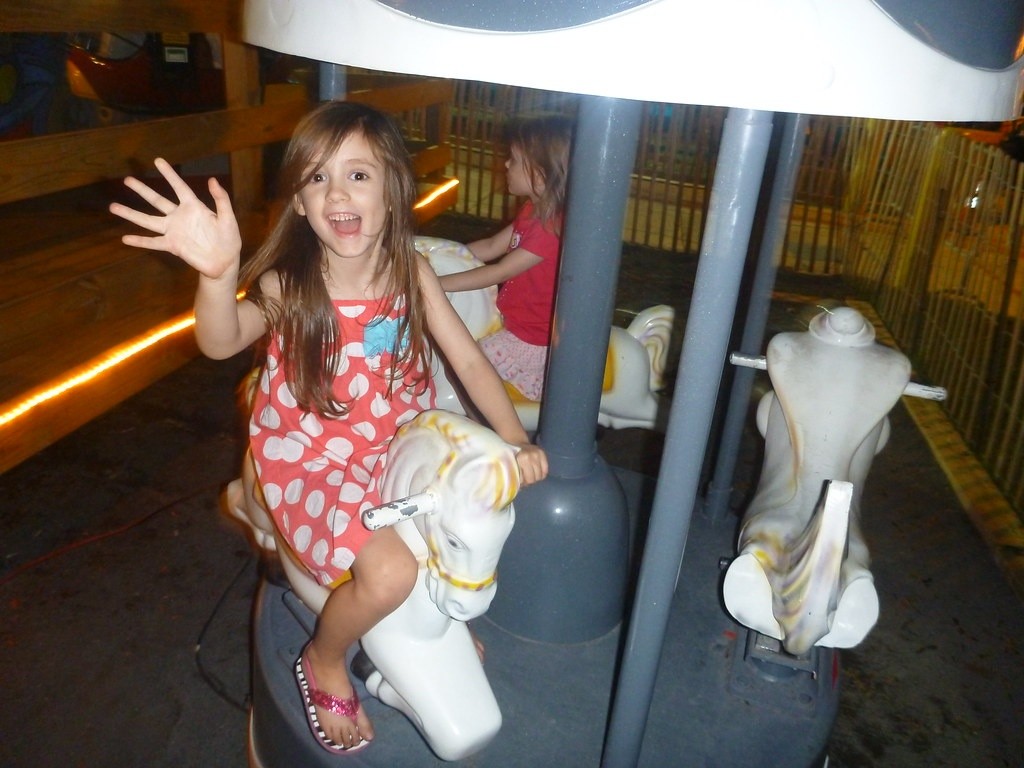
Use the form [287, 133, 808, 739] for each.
[110, 102, 551, 755]
[435, 113, 573, 401]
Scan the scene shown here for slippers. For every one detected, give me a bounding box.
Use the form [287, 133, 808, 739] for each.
[294, 644, 374, 752]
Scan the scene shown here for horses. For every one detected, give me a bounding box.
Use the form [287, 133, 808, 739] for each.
[223, 366, 521, 760]
[717, 307, 914, 657]
[402, 233, 687, 430]
